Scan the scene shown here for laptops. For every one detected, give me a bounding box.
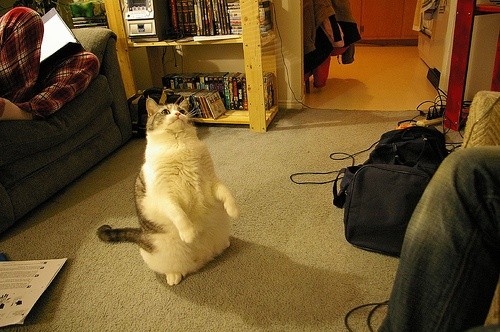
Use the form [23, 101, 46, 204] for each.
[39, 7, 81, 69]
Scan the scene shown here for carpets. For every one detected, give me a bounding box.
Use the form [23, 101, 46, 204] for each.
[0, 107, 463, 332]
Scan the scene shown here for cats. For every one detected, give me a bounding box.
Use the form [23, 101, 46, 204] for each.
[96, 96, 239, 286]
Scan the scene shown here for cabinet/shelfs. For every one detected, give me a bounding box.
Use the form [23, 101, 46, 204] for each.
[348, 0, 418, 41]
[104, 0, 278, 133]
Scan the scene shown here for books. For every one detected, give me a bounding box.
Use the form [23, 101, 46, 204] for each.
[170, 0, 271, 35]
[162, 73, 274, 120]
[41, 7, 86, 71]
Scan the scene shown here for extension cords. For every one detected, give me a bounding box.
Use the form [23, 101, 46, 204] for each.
[416, 115, 444, 127]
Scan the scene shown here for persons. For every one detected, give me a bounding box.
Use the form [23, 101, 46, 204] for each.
[375, 145, 500, 332]
[0, 7, 103, 122]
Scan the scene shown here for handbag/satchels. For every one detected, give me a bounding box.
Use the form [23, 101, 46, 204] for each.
[332, 125, 449, 257]
[128, 85, 197, 136]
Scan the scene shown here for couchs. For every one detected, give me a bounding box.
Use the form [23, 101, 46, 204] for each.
[0, 28, 133, 233]
